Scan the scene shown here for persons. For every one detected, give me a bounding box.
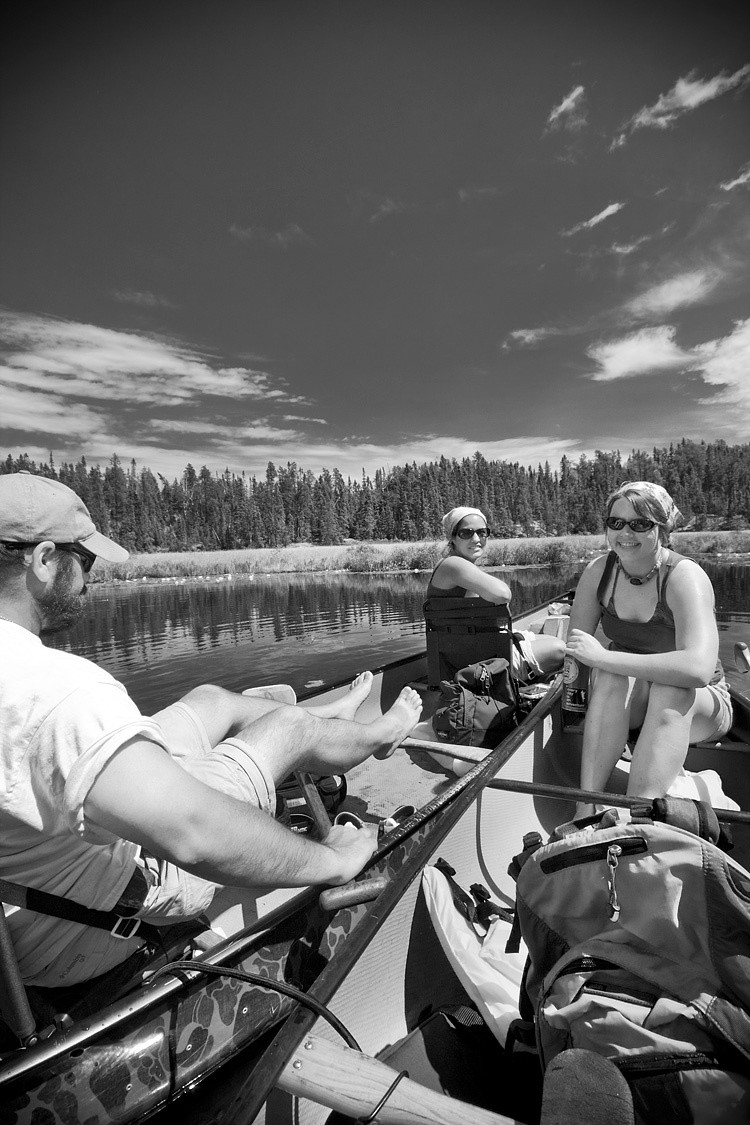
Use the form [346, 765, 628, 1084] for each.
[0, 468, 424, 993]
[568, 482, 733, 823]
[422, 506, 567, 683]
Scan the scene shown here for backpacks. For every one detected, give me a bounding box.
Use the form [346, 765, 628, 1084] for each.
[503, 795, 750, 1125]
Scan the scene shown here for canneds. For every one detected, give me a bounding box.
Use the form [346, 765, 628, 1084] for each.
[561, 652, 590, 713]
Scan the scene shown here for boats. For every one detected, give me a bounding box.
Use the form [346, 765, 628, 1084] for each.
[0, 583, 583, 1125]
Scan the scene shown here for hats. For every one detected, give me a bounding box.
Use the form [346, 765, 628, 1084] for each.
[0, 469, 130, 564]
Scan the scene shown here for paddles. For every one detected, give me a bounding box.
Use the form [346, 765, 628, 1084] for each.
[242, 683, 387, 911]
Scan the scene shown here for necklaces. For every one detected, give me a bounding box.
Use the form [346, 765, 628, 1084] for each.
[619, 558, 660, 586]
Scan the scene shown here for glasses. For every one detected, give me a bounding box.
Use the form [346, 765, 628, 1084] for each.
[4, 541, 96, 571]
[457, 528, 491, 539]
[606, 516, 658, 532]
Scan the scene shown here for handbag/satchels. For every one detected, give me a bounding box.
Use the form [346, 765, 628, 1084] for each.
[432, 658, 528, 750]
